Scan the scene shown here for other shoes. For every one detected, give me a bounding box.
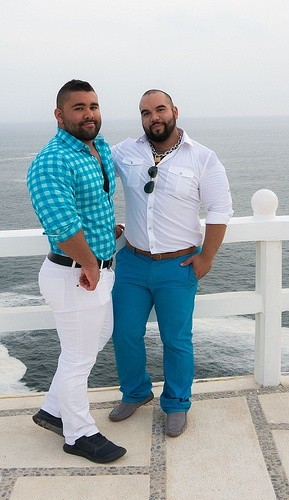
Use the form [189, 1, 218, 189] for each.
[165, 408, 186, 436]
[109, 391, 154, 421]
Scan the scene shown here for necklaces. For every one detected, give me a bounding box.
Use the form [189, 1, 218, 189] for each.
[149, 131, 182, 163]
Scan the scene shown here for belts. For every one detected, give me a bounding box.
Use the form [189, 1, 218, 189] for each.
[126, 239, 196, 261]
[48, 252, 113, 271]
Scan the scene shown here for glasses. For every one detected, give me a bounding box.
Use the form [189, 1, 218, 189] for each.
[143, 165, 158, 194]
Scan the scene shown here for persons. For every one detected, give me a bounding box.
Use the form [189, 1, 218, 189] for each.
[109, 89, 235, 438]
[33, 78, 127, 463]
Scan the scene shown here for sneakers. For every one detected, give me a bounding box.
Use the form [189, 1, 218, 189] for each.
[63, 433, 126, 463]
[32, 411, 64, 436]
